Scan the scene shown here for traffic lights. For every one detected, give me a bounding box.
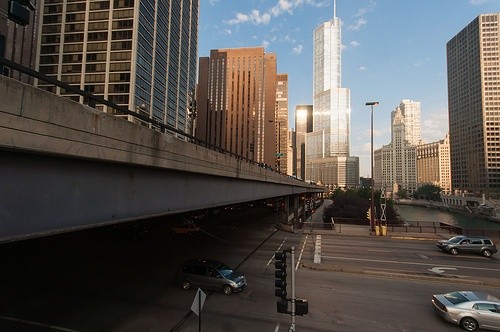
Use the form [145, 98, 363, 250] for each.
[274, 252, 288, 314]
[295, 300, 309, 316]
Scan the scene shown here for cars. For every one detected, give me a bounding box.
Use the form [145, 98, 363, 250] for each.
[181, 262, 248, 295]
[168, 224, 200, 236]
[437, 235, 497, 257]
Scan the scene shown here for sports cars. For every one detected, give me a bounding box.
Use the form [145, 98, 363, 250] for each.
[429, 290, 500, 332]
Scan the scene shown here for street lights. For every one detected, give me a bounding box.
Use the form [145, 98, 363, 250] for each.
[268, 120, 280, 171]
[366, 102, 378, 231]
